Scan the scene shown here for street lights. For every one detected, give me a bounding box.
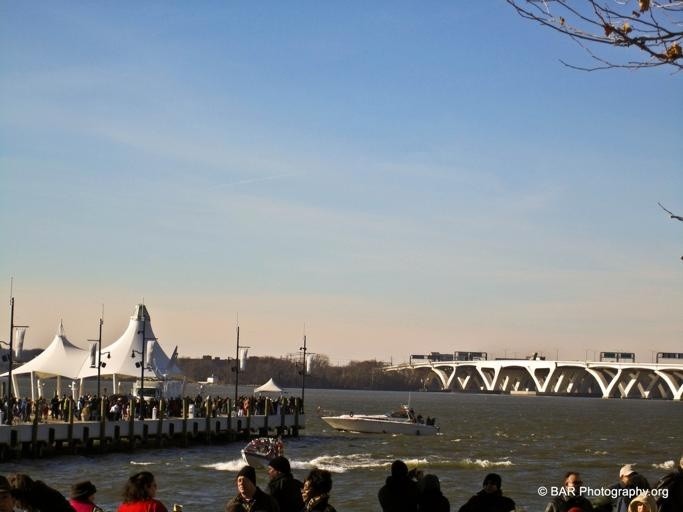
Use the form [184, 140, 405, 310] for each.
[86, 319, 109, 418]
[0, 296, 29, 424]
[293, 335, 315, 416]
[230, 325, 249, 410]
[130, 317, 146, 420]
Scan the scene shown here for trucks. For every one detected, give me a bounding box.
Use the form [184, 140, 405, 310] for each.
[131, 378, 182, 403]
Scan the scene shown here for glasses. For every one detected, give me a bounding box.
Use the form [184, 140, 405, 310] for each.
[566, 481, 581, 485]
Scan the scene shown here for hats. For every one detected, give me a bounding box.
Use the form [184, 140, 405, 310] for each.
[236, 466, 255, 486]
[482, 473, 502, 489]
[69, 480, 97, 498]
[268, 456, 289, 473]
[391, 459, 408, 478]
[619, 464, 636, 478]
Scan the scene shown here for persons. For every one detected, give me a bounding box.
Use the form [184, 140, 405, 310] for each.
[262, 455, 304, 510]
[0, 391, 305, 426]
[400, 406, 436, 426]
[225, 466, 271, 511]
[544, 456, 683, 512]
[0, 469, 170, 512]
[248, 435, 286, 457]
[300, 468, 336, 511]
[376, 460, 516, 512]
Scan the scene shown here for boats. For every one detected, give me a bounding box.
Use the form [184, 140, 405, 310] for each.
[241, 436, 284, 469]
[320, 404, 439, 437]
